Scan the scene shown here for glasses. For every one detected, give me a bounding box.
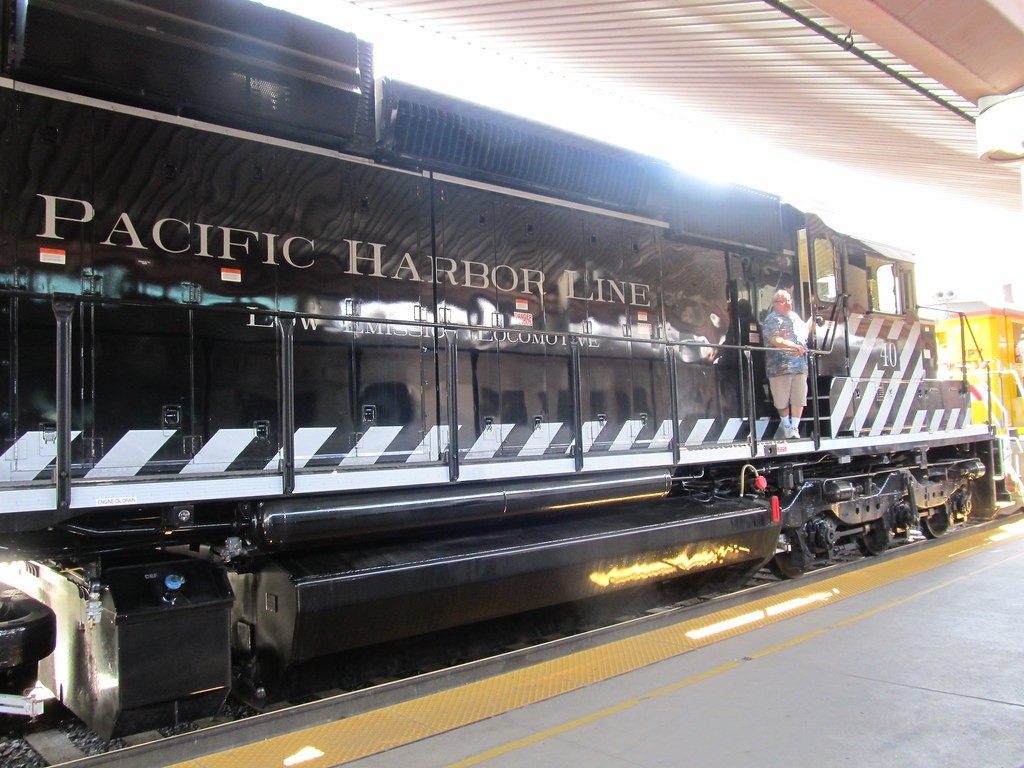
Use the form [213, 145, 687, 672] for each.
[775, 299, 792, 304]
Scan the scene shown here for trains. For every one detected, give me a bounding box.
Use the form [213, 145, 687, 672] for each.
[0, 0, 1000, 734]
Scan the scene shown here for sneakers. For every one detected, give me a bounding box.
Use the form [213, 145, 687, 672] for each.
[790, 426, 800, 438]
[784, 425, 791, 438]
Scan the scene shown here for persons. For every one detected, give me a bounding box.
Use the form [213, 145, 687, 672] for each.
[761, 288, 814, 441]
